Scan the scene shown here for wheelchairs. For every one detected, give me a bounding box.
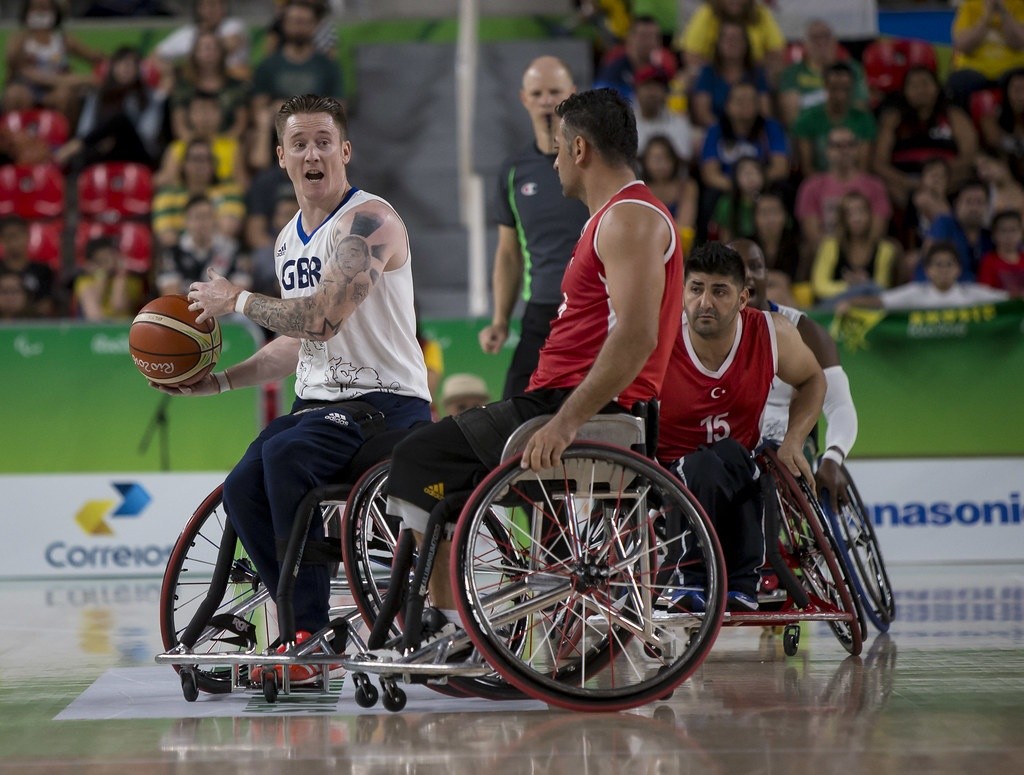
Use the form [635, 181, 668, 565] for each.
[155, 402, 528, 703]
[553, 447, 868, 659]
[342, 396, 728, 712]
[644, 455, 896, 632]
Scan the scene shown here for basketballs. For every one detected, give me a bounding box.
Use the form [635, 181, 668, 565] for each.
[128, 294, 223, 391]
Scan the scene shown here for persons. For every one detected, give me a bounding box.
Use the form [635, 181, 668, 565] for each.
[145, 94, 434, 685]
[656, 239, 859, 611]
[476, 54, 590, 612]
[582, 0, 1024, 304]
[0, 0, 337, 323]
[414, 291, 490, 421]
[383, 85, 685, 665]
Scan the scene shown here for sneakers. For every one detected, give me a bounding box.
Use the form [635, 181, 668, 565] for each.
[725, 590, 759, 611]
[251, 632, 349, 684]
[667, 586, 705, 612]
[354, 605, 473, 661]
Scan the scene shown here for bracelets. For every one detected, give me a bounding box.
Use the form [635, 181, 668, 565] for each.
[213, 370, 232, 395]
[821, 449, 844, 468]
[234, 289, 253, 315]
